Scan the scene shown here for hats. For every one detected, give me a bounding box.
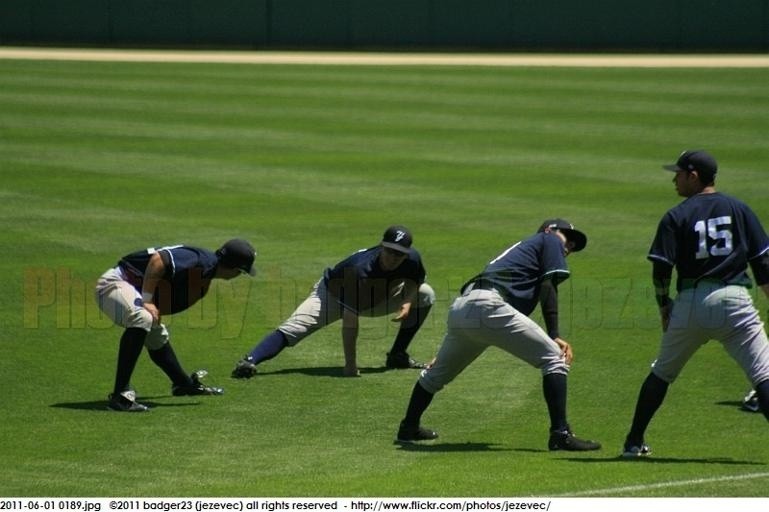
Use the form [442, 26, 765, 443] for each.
[380, 224, 414, 256]
[215, 239, 257, 277]
[659, 148, 720, 173]
[536, 217, 587, 252]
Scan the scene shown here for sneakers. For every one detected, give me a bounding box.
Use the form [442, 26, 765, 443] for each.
[547, 430, 600, 453]
[397, 423, 439, 440]
[108, 391, 147, 413]
[622, 445, 652, 459]
[171, 370, 222, 396]
[228, 356, 257, 378]
[386, 352, 430, 370]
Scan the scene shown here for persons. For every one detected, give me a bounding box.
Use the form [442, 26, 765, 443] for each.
[94, 238, 258, 413]
[234, 223, 437, 379]
[395, 216, 602, 452]
[619, 148, 769, 457]
